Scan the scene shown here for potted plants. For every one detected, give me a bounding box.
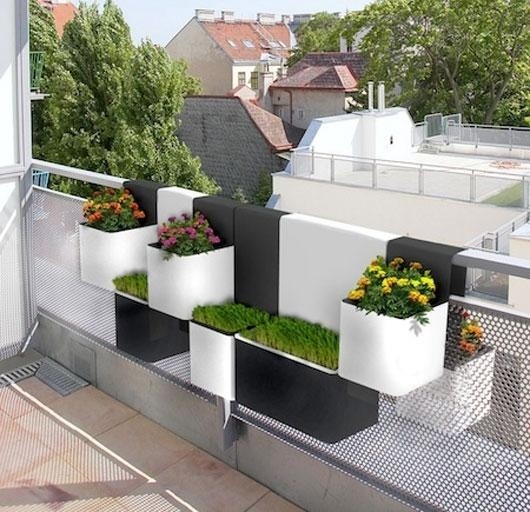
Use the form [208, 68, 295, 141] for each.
[190, 305, 269, 402]
[234, 314, 378, 443]
[112, 271, 189, 363]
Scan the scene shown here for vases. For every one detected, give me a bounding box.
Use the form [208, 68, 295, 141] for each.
[337, 297, 449, 397]
[77, 223, 159, 294]
[395, 347, 496, 438]
[146, 240, 234, 321]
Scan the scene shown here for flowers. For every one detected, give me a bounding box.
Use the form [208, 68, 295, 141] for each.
[445, 303, 484, 369]
[347, 253, 436, 324]
[156, 211, 221, 266]
[81, 188, 145, 232]
